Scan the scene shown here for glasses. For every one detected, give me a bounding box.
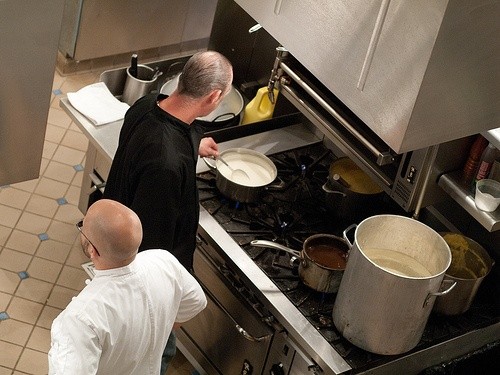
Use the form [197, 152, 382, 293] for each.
[76, 221, 100, 256]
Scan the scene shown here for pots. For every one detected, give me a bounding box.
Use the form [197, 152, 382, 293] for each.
[250, 233, 352, 295]
[203, 147, 286, 202]
[320, 157, 390, 207]
[160, 62, 245, 129]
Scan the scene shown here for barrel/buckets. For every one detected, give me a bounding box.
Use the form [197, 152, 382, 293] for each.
[331, 214, 458, 356]
[438, 231, 493, 317]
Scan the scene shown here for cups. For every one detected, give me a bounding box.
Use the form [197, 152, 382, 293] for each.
[474, 179, 500, 213]
[122, 63, 157, 107]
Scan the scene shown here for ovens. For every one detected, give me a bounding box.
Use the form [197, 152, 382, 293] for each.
[173, 244, 274, 375]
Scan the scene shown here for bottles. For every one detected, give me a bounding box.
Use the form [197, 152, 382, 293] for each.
[463, 136, 500, 183]
[240, 81, 280, 126]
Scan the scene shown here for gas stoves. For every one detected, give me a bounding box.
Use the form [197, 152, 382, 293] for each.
[188, 142, 500, 375]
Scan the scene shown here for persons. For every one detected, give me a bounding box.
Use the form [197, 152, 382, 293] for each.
[48, 199, 207, 375]
[101, 51, 233, 375]
